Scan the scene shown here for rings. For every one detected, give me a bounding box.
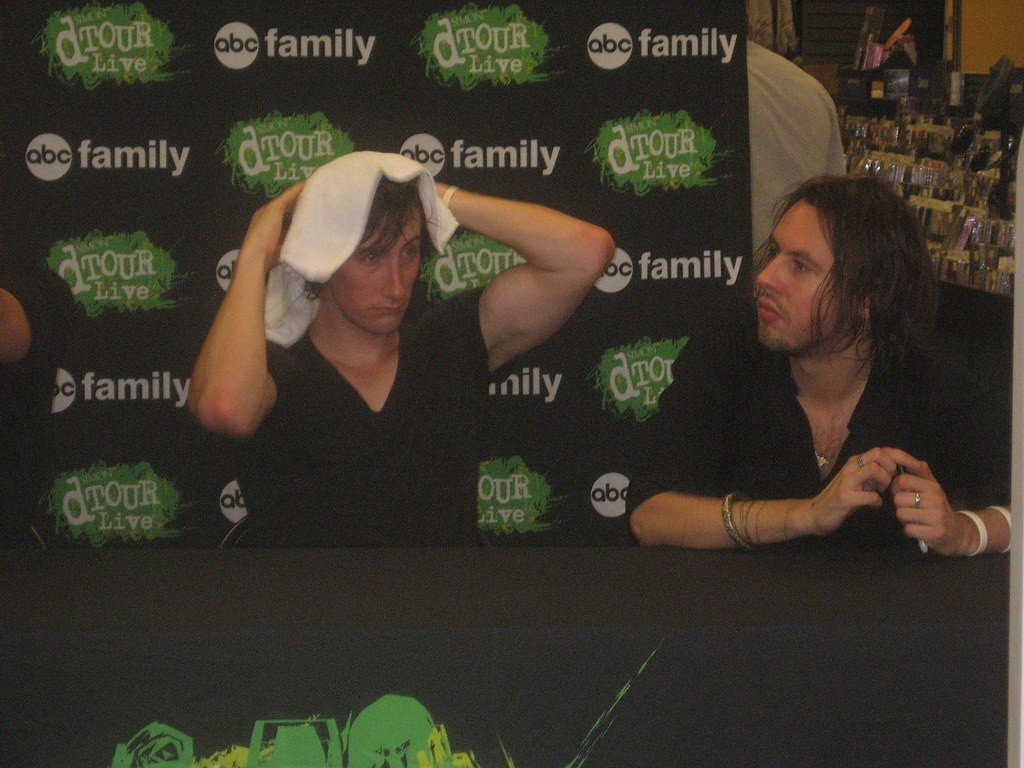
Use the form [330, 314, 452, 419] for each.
[915, 491, 920, 508]
[857, 455, 865, 467]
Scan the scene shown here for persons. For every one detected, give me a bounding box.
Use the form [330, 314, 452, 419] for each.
[626, 177, 1014, 558]
[186, 152, 615, 547]
[0, 265, 74, 546]
[747, 38, 847, 281]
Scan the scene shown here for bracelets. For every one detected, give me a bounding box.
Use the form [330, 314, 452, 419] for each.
[445, 186, 457, 205]
[986, 505, 1012, 553]
[722, 489, 753, 550]
[957, 510, 988, 556]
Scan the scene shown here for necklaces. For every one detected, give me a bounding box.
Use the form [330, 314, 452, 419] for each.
[814, 451, 838, 467]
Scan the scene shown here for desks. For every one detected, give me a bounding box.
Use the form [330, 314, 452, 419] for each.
[0, 539, 1008, 768]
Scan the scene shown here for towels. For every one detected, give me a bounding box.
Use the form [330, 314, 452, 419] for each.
[260, 149, 460, 347]
